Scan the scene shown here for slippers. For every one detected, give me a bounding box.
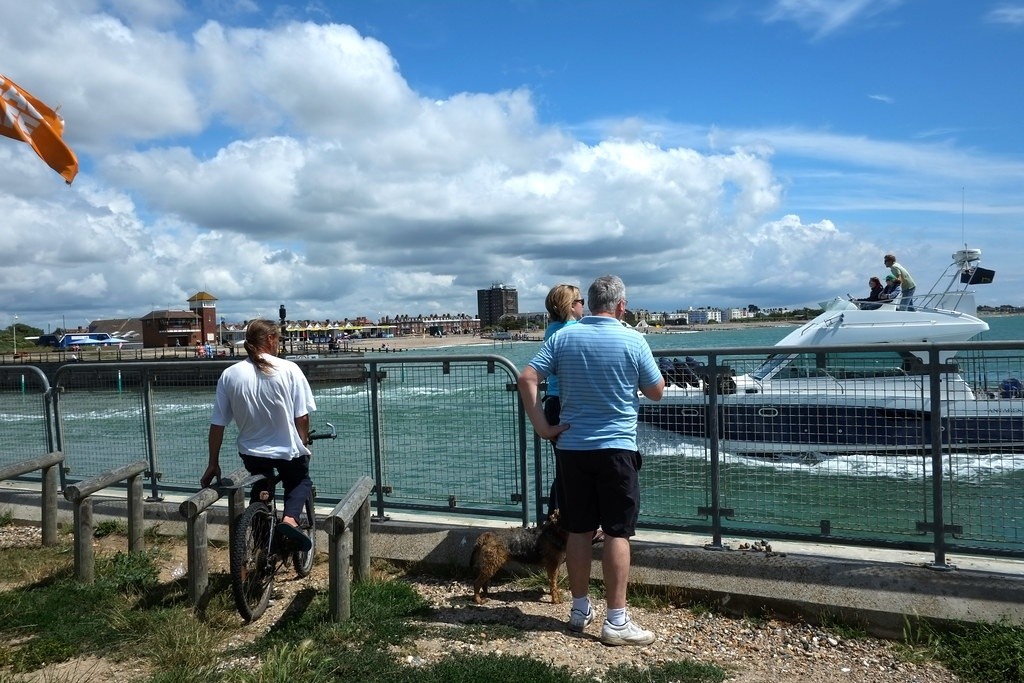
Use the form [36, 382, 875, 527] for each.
[279, 522, 311, 549]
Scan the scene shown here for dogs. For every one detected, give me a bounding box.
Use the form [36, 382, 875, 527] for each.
[467, 507, 598, 606]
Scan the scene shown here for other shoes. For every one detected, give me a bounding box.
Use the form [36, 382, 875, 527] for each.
[591, 531, 606, 543]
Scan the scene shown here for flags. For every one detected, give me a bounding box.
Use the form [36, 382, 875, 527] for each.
[0, 74, 79, 185]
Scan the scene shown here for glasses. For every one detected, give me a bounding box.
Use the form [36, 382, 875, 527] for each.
[574, 298, 584, 305]
[886, 279, 890, 282]
[618, 299, 628, 305]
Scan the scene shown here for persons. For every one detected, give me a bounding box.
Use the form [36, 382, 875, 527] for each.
[72, 345, 79, 352]
[197, 318, 318, 552]
[317, 337, 341, 354]
[849, 255, 916, 311]
[515, 275, 665, 646]
[175, 338, 180, 347]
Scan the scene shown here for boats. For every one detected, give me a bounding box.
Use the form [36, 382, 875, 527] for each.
[634, 184, 1023, 460]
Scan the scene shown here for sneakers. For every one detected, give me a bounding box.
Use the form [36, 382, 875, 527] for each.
[602, 612, 655, 645]
[569, 606, 596, 631]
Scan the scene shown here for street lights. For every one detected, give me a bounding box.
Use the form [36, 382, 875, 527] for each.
[12, 313, 18, 354]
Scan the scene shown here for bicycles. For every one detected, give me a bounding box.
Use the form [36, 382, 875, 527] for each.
[217, 423, 339, 623]
